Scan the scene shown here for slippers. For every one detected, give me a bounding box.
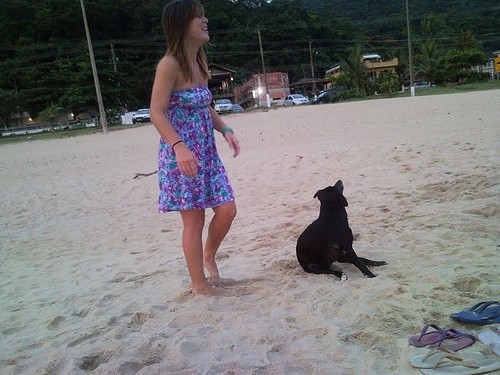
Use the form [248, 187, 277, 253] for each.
[409, 323, 462, 347]
[429, 329, 479, 352]
[411, 346, 486, 369]
[420, 356, 500, 375]
[479, 331, 500, 356]
[451, 301, 500, 325]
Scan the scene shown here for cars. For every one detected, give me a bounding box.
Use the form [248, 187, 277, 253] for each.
[283, 94, 310, 106]
[214, 99, 233, 114]
[315, 89, 344, 104]
[233, 104, 244, 112]
[132, 108, 150, 124]
[409, 82, 429, 88]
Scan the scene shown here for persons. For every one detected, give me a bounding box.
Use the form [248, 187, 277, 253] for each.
[150, 0, 239, 298]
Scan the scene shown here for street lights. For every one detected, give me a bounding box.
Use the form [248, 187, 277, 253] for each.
[308, 39, 319, 94]
[256, 24, 271, 109]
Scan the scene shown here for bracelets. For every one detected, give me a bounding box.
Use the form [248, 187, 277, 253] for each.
[223, 127, 235, 137]
[172, 140, 183, 149]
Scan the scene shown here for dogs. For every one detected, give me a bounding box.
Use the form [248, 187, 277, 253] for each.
[295, 178, 386, 279]
